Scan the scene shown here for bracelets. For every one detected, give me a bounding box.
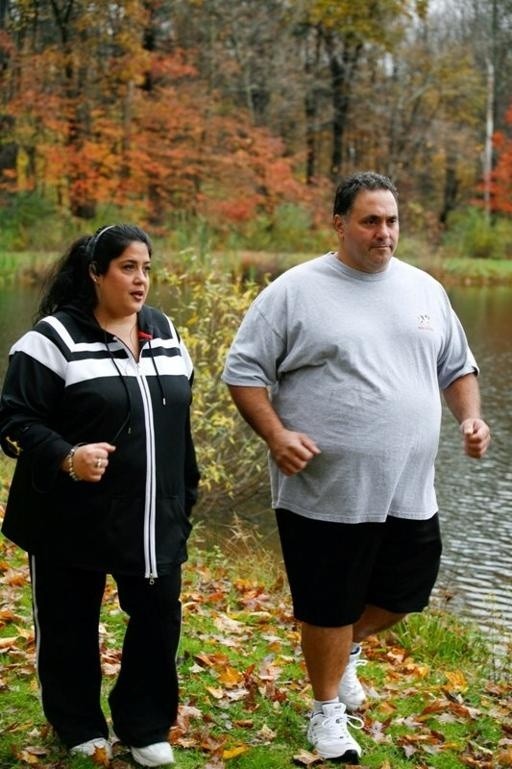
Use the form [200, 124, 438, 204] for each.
[69, 443, 84, 482]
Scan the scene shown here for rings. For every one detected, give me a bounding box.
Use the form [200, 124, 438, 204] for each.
[96, 456, 102, 469]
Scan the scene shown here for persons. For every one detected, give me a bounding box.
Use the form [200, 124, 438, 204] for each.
[1, 224, 200, 768]
[217, 170, 491, 764]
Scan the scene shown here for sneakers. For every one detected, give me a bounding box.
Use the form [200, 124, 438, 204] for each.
[131, 742, 175, 768]
[70, 738, 114, 761]
[306, 702, 365, 765]
[339, 647, 368, 712]
